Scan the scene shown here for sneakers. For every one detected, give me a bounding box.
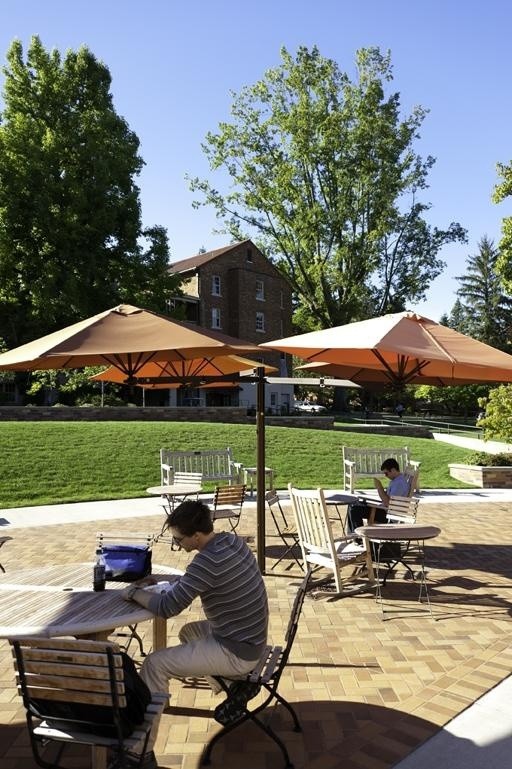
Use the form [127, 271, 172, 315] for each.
[214, 681, 261, 724]
[108, 748, 158, 769]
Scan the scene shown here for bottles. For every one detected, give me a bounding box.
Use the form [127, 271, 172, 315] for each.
[93, 550, 106, 592]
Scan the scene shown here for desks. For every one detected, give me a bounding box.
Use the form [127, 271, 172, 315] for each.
[244, 466, 276, 499]
[354, 522, 441, 622]
[0, 559, 191, 766]
[144, 484, 205, 553]
[296, 489, 359, 558]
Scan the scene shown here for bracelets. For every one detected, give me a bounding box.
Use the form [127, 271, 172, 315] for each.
[127, 586, 143, 600]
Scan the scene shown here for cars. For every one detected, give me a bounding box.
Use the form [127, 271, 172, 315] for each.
[296, 400, 327, 413]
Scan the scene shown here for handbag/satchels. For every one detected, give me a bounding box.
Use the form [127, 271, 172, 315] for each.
[96, 545, 152, 582]
[26, 651, 152, 740]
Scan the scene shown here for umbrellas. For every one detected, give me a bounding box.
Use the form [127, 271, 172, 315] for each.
[86, 354, 280, 394]
[257, 312, 512, 385]
[0, 303, 276, 392]
[290, 361, 498, 389]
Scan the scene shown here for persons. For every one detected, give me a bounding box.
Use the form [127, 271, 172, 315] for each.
[99, 499, 271, 769]
[396, 403, 403, 418]
[345, 458, 410, 548]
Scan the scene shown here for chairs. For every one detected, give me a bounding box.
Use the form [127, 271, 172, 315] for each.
[89, 530, 157, 661]
[396, 461, 419, 497]
[263, 487, 334, 574]
[284, 483, 384, 598]
[170, 470, 202, 503]
[385, 494, 420, 535]
[208, 483, 248, 542]
[3, 636, 173, 767]
[181, 568, 316, 764]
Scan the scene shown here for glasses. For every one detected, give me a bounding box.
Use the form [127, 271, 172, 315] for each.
[384, 471, 389, 476]
[172, 535, 187, 547]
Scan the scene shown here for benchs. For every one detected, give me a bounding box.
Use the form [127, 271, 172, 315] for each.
[343, 446, 422, 494]
[160, 447, 243, 498]
